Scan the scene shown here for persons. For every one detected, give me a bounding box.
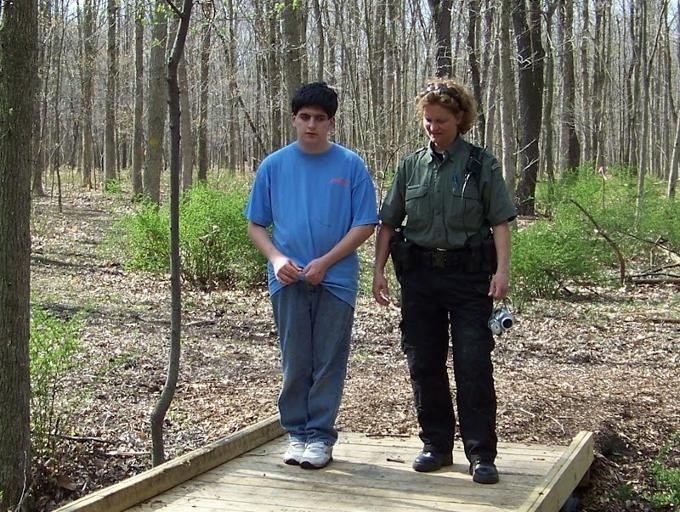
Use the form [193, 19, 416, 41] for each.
[369, 73, 520, 486]
[246, 78, 380, 472]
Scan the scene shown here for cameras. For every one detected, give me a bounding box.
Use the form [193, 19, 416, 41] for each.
[486, 308, 516, 336]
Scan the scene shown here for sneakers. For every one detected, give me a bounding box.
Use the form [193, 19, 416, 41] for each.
[300, 442, 332, 469]
[470, 461, 498, 483]
[413, 452, 453, 471]
[283, 441, 305, 465]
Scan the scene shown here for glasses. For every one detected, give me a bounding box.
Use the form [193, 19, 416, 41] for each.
[426, 82, 460, 98]
